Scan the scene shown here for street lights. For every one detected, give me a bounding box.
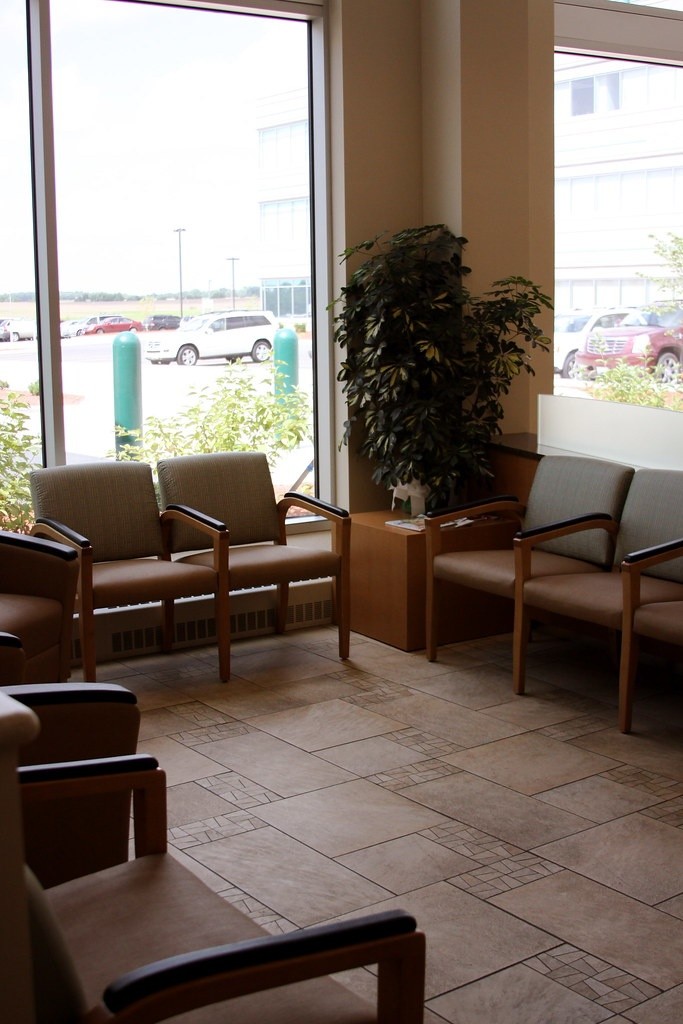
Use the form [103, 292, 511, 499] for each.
[224, 256, 241, 309]
[171, 226, 187, 320]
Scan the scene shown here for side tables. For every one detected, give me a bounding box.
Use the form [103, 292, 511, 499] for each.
[332, 508, 515, 652]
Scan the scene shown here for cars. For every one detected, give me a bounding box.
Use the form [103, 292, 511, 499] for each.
[0, 315, 112, 342]
[146, 315, 181, 331]
[554, 298, 683, 385]
[83, 317, 143, 335]
[178, 312, 224, 330]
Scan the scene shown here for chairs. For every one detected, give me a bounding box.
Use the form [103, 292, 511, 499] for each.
[0, 684, 429, 1024]
[29, 452, 351, 683]
[425, 455, 683, 734]
[0, 530, 80, 685]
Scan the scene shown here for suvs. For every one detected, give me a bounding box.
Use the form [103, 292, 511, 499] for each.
[144, 310, 280, 367]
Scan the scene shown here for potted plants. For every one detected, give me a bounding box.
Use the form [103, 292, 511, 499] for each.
[326, 223, 555, 517]
